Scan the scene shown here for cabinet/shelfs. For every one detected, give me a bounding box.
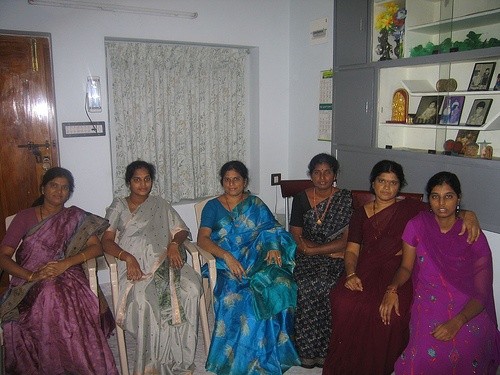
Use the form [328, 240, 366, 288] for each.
[331, 0, 500, 234]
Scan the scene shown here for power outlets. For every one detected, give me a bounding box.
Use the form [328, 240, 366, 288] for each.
[271, 173, 282, 186]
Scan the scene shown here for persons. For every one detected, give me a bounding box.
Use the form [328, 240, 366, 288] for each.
[411, 68, 490, 125]
[378, 171, 500, 375]
[101, 160, 204, 375]
[289, 153, 355, 369]
[197, 160, 301, 375]
[0, 167, 120, 375]
[322, 160, 481, 375]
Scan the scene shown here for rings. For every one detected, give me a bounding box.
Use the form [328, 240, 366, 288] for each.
[278, 256, 280, 259]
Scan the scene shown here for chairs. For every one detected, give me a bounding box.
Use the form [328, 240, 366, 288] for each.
[102, 222, 211, 375]
[277, 177, 312, 235]
[349, 189, 426, 213]
[5, 211, 98, 302]
[192, 194, 219, 290]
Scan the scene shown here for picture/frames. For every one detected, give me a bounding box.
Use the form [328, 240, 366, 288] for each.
[468, 61, 495, 90]
[411, 95, 444, 124]
[464, 98, 494, 127]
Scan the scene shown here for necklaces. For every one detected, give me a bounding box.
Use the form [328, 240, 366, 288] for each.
[40, 204, 44, 221]
[224, 194, 244, 211]
[373, 197, 397, 215]
[313, 186, 335, 226]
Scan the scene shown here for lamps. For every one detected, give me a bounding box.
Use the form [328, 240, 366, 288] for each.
[28, 0, 199, 21]
[86, 75, 103, 112]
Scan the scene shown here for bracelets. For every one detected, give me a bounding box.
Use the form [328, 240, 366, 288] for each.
[331, 253, 333, 257]
[80, 252, 86, 263]
[459, 312, 468, 324]
[118, 250, 125, 260]
[385, 292, 398, 294]
[27, 271, 34, 283]
[345, 273, 355, 278]
[170, 241, 179, 247]
[386, 288, 398, 291]
